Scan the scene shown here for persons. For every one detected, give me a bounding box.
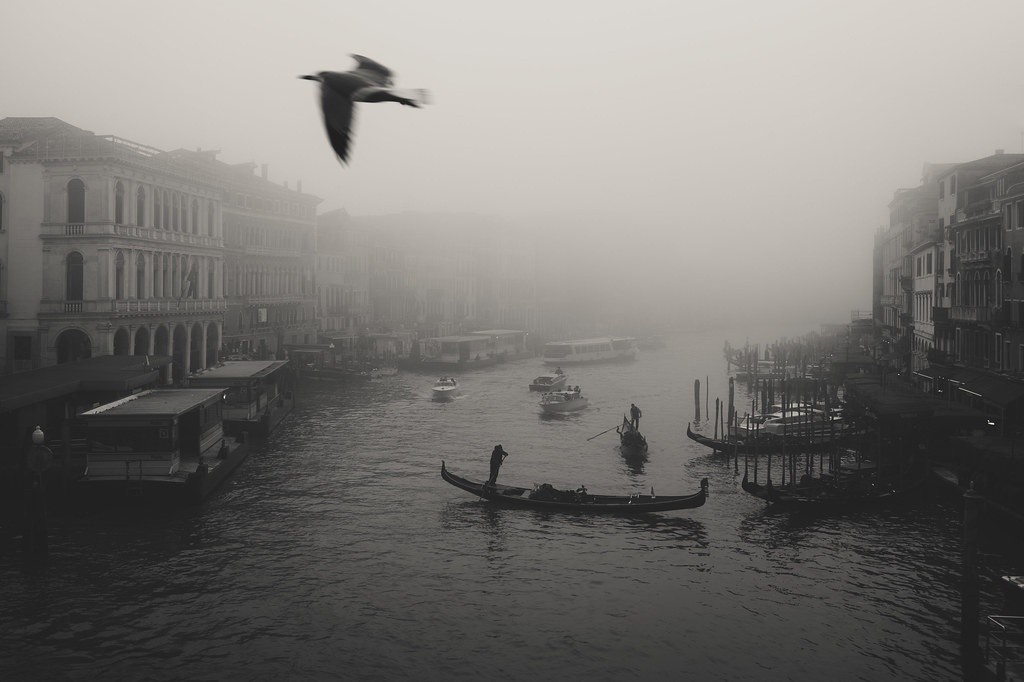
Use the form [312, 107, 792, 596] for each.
[489, 445, 508, 486]
[555, 366, 563, 374]
[630, 403, 642, 431]
[439, 375, 456, 386]
[542, 385, 581, 402]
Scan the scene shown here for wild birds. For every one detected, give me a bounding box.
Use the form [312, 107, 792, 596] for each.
[297, 53, 435, 167]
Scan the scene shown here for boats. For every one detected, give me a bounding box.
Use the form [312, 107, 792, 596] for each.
[75, 358, 295, 509]
[440, 459, 712, 515]
[686, 399, 850, 456]
[528, 366, 568, 392]
[419, 328, 531, 372]
[537, 385, 589, 413]
[432, 376, 460, 398]
[615, 415, 648, 456]
[543, 336, 640, 366]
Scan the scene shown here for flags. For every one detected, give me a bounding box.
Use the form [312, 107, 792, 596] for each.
[187, 259, 197, 299]
